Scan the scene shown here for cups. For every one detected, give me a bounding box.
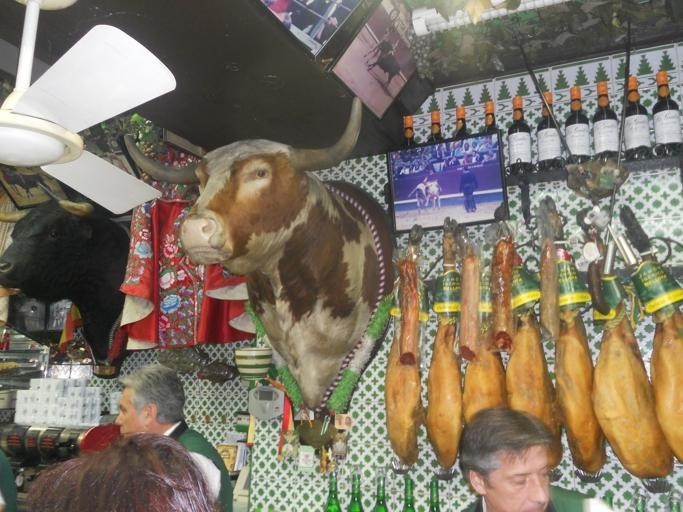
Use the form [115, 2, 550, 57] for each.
[233, 347, 271, 380]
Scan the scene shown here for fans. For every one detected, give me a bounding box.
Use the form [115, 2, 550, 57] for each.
[0, 0, 178, 217]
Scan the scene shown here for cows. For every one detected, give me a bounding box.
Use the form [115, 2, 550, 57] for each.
[362, 39, 400, 90]
[122, 97, 397, 413]
[0, 182, 131, 366]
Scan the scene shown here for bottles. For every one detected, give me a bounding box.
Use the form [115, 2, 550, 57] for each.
[324, 471, 442, 511]
[401, 65, 683, 162]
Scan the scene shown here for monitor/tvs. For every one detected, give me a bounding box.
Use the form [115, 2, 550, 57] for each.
[385, 131, 510, 235]
[328, 0, 423, 121]
[253, 0, 365, 58]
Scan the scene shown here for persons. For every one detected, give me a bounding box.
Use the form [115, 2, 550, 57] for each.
[28, 435, 216, 511]
[362, 27, 395, 65]
[388, 134, 500, 215]
[313, 14, 341, 45]
[111, 362, 232, 511]
[457, 407, 615, 512]
